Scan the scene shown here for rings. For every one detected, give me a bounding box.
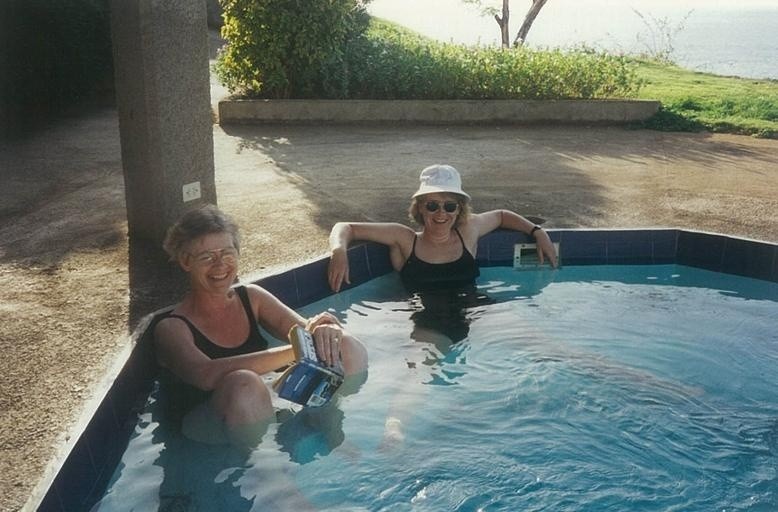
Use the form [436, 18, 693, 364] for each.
[330, 337, 339, 343]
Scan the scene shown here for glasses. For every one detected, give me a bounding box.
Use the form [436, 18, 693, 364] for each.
[423, 200, 460, 215]
[188, 246, 239, 266]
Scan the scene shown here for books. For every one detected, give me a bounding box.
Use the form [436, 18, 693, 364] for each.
[270, 325, 344, 408]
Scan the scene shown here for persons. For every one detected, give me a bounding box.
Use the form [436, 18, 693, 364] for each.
[328, 163, 607, 458]
[154, 204, 368, 462]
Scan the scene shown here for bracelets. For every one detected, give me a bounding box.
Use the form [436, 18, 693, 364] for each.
[531, 224, 542, 237]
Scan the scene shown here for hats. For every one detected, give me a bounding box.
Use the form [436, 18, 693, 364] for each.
[411, 164, 471, 207]
